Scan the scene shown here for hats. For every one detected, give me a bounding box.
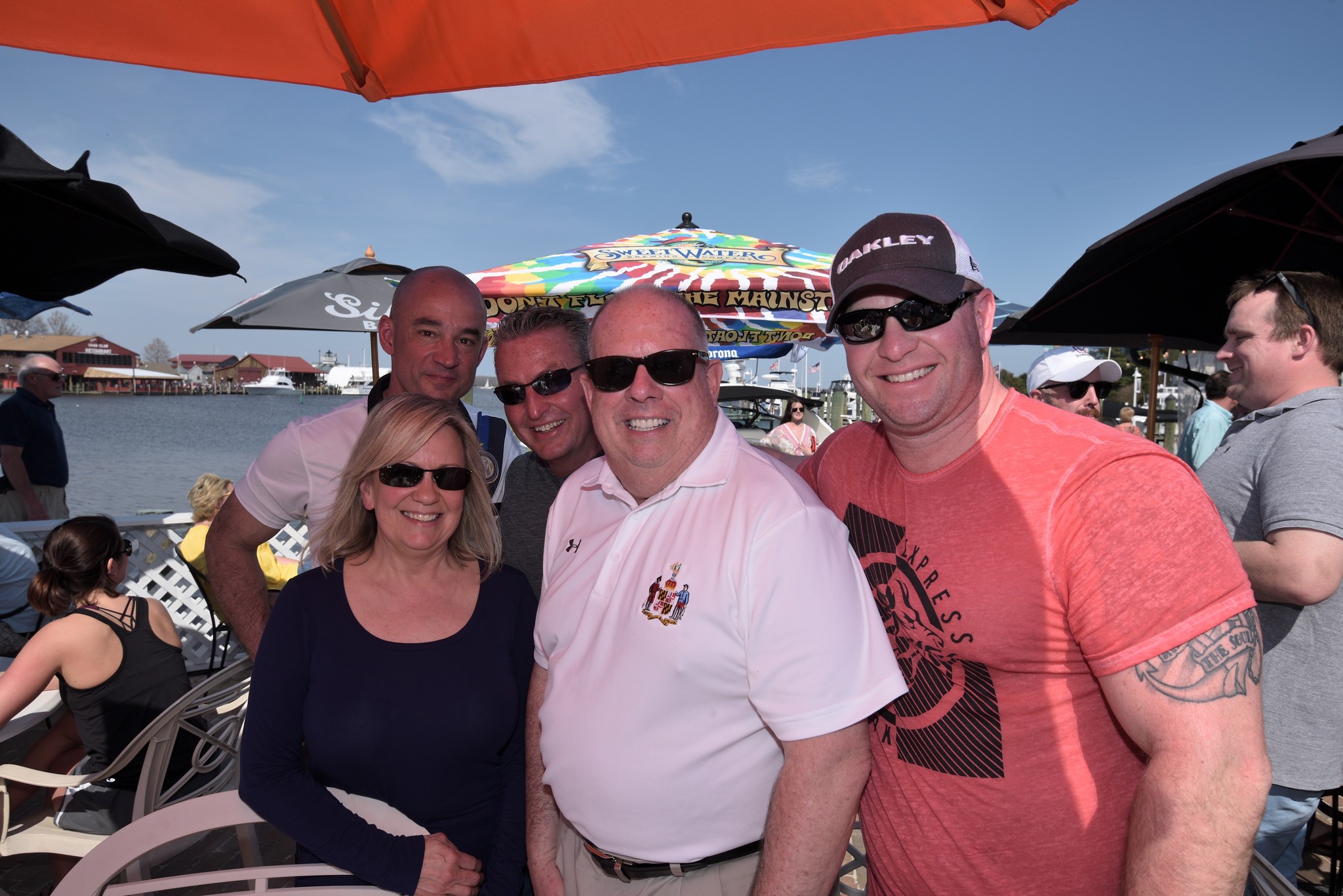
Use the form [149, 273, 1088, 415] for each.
[1026, 346, 1122, 395]
[825, 213, 990, 334]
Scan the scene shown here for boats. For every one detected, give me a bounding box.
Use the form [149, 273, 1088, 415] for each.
[1114, 351, 1232, 456]
[341, 379, 375, 395]
[723, 352, 881, 444]
[240, 356, 304, 396]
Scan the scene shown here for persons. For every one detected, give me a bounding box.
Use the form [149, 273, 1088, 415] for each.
[0, 211, 1343, 896]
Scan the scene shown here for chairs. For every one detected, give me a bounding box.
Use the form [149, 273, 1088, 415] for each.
[45, 781, 433, 896]
[173, 539, 238, 680]
[0, 655, 268, 896]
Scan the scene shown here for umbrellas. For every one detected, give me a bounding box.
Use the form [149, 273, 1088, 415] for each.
[0, 1, 1343, 443]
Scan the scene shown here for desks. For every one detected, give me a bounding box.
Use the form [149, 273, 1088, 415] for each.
[0, 656, 64, 744]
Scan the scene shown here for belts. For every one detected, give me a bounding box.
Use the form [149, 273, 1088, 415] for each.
[580, 838, 768, 883]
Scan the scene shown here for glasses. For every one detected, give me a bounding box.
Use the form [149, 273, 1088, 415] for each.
[379, 463, 472, 491]
[112, 539, 133, 557]
[493, 364, 587, 406]
[791, 408, 804, 413]
[1256, 272, 1317, 329]
[585, 349, 711, 392]
[31, 373, 62, 381]
[1038, 380, 1114, 399]
[835, 289, 999, 344]
